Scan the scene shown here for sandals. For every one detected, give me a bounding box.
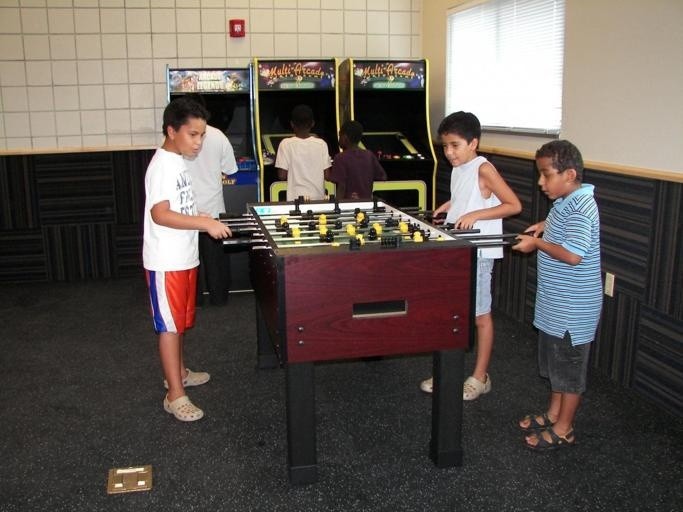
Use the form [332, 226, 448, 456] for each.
[525, 426, 576, 451]
[164, 368, 210, 389]
[421, 378, 434, 393]
[164, 394, 203, 422]
[463, 373, 491, 401]
[519, 412, 556, 431]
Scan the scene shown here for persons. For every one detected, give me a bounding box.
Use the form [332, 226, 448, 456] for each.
[325, 121, 388, 202]
[419, 110, 524, 402]
[511, 139, 603, 451]
[143, 98, 234, 420]
[275, 104, 332, 202]
[194, 123, 240, 305]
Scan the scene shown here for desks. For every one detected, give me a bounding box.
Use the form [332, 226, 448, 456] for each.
[243, 195, 479, 489]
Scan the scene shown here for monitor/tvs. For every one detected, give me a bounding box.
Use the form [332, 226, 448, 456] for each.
[265, 133, 316, 153]
[358, 131, 420, 156]
[227, 134, 251, 161]
